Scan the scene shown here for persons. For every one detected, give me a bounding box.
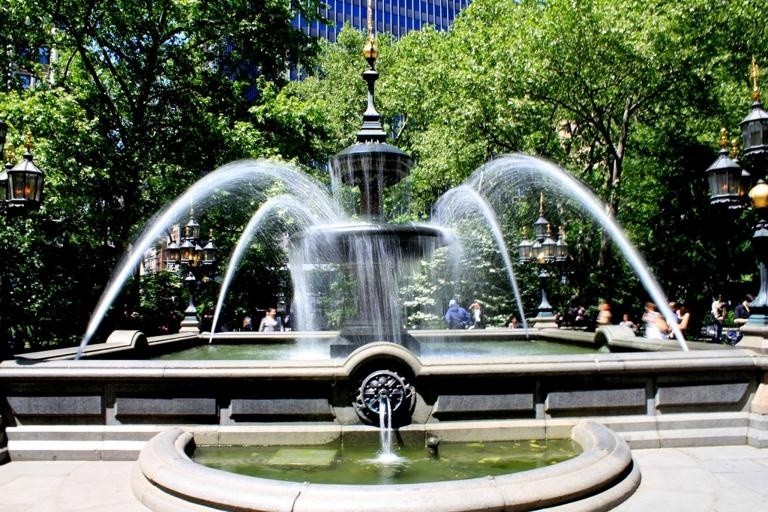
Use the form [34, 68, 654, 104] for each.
[596, 293, 754, 344]
[468, 303, 484, 329]
[445, 299, 470, 329]
[243, 308, 292, 331]
[508, 316, 519, 328]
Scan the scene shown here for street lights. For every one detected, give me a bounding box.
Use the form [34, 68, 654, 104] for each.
[706, 55, 768, 336]
